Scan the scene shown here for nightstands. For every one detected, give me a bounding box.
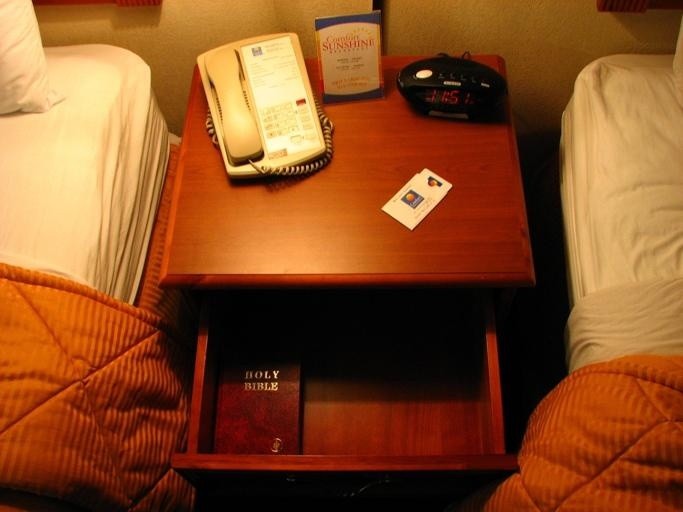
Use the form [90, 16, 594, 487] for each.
[157, 52, 538, 471]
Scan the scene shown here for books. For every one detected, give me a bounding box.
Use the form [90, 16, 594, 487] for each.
[214, 358, 303, 457]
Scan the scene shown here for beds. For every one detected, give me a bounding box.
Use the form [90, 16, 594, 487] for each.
[0, 43, 194, 512]
[479, 53, 682, 512]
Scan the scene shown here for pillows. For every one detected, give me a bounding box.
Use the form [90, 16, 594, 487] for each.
[0, 0, 68, 115]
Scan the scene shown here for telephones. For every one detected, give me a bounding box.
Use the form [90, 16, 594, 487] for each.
[196, 31, 335, 180]
